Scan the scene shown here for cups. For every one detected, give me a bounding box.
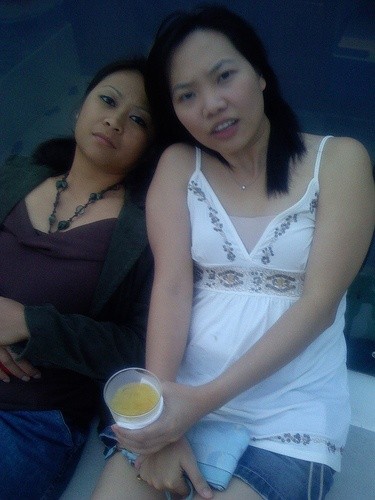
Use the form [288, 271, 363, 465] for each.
[103, 368, 163, 430]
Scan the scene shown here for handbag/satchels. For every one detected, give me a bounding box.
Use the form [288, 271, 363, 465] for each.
[104, 420, 250, 500]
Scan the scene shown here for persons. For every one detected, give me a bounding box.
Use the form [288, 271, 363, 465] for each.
[0, 58, 185, 500]
[90, 4, 374, 500]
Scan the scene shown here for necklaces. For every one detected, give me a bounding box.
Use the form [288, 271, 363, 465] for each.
[47, 171, 125, 234]
[226, 164, 269, 191]
